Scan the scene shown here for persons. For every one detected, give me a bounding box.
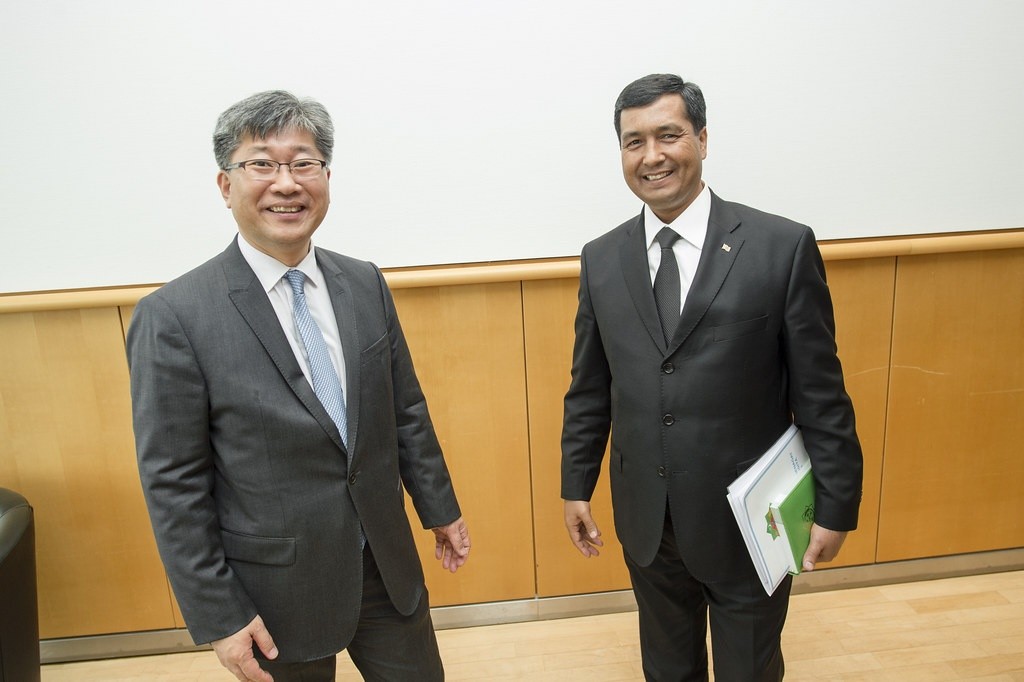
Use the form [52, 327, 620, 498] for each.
[560, 74, 864, 682]
[125, 91, 471, 682]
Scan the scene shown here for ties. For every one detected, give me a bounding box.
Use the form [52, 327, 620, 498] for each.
[653, 226, 681, 348]
[282, 269, 348, 450]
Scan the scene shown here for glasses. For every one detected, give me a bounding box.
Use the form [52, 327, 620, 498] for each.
[224, 158, 328, 181]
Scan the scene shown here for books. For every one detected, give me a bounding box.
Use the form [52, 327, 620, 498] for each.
[769, 457, 816, 575]
